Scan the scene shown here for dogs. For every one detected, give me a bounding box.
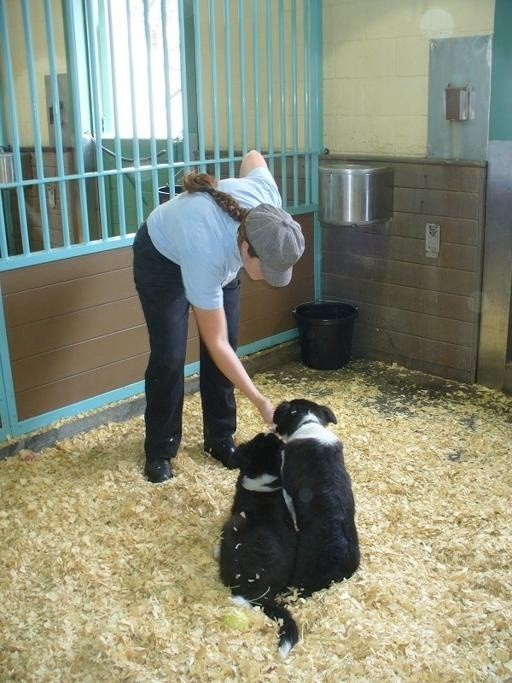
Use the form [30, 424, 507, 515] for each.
[211, 431, 301, 659]
[225, 397, 361, 610]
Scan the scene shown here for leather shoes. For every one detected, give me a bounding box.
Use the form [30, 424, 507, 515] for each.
[142, 455, 176, 482]
[202, 437, 239, 471]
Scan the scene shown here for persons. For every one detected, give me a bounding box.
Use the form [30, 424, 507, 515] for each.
[128, 147, 305, 482]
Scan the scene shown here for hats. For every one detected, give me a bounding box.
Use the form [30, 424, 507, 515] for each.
[242, 199, 308, 291]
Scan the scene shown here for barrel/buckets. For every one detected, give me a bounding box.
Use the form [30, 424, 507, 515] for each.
[158, 168, 185, 205]
[291, 297, 358, 370]
